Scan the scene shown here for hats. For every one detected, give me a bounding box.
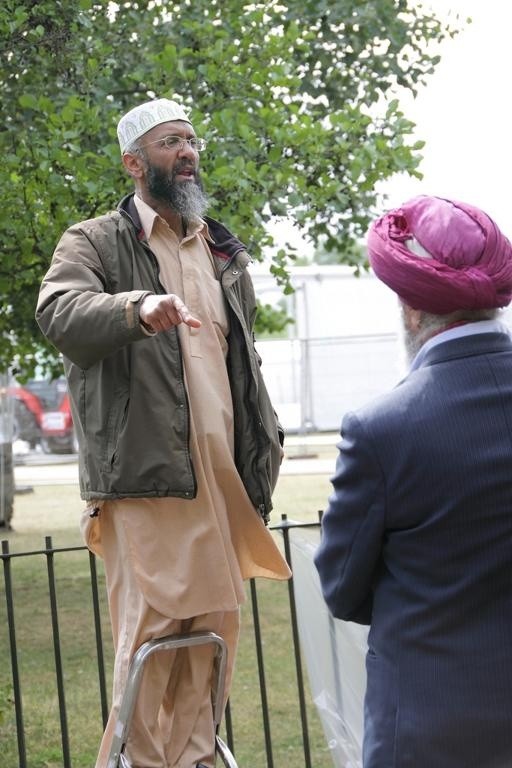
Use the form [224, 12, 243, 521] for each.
[117, 98, 192, 153]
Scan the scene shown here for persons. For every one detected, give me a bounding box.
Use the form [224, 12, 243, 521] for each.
[35, 98, 286, 768]
[313, 193, 512, 768]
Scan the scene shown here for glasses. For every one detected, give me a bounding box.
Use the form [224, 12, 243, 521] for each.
[136, 136, 208, 152]
